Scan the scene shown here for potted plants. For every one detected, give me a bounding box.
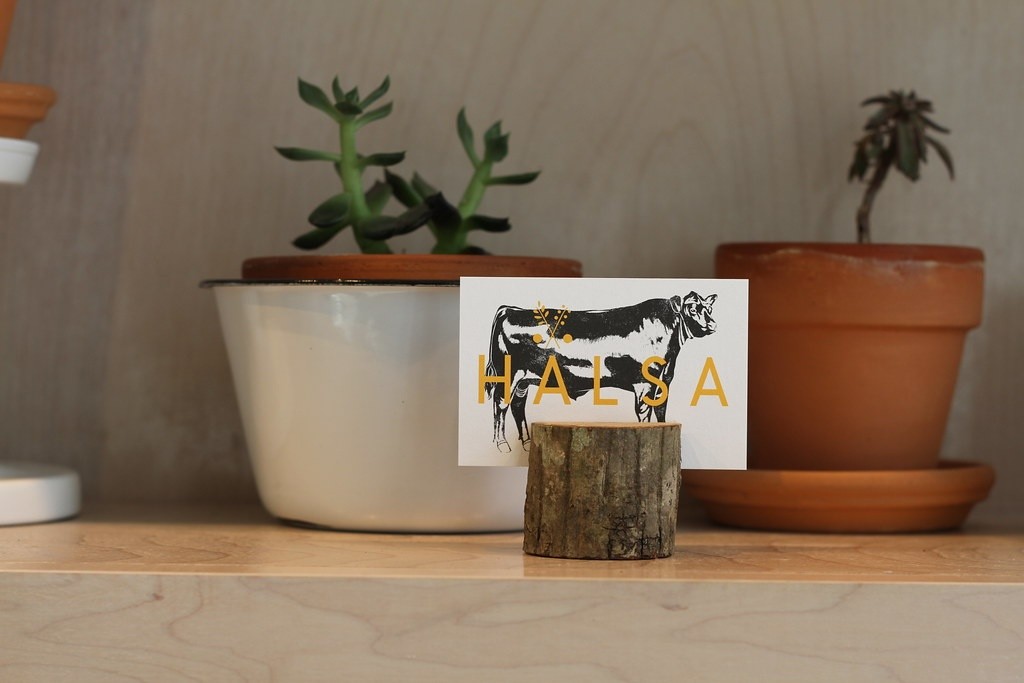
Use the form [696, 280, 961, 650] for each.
[712, 89, 985, 468]
[198, 76, 586, 534]
